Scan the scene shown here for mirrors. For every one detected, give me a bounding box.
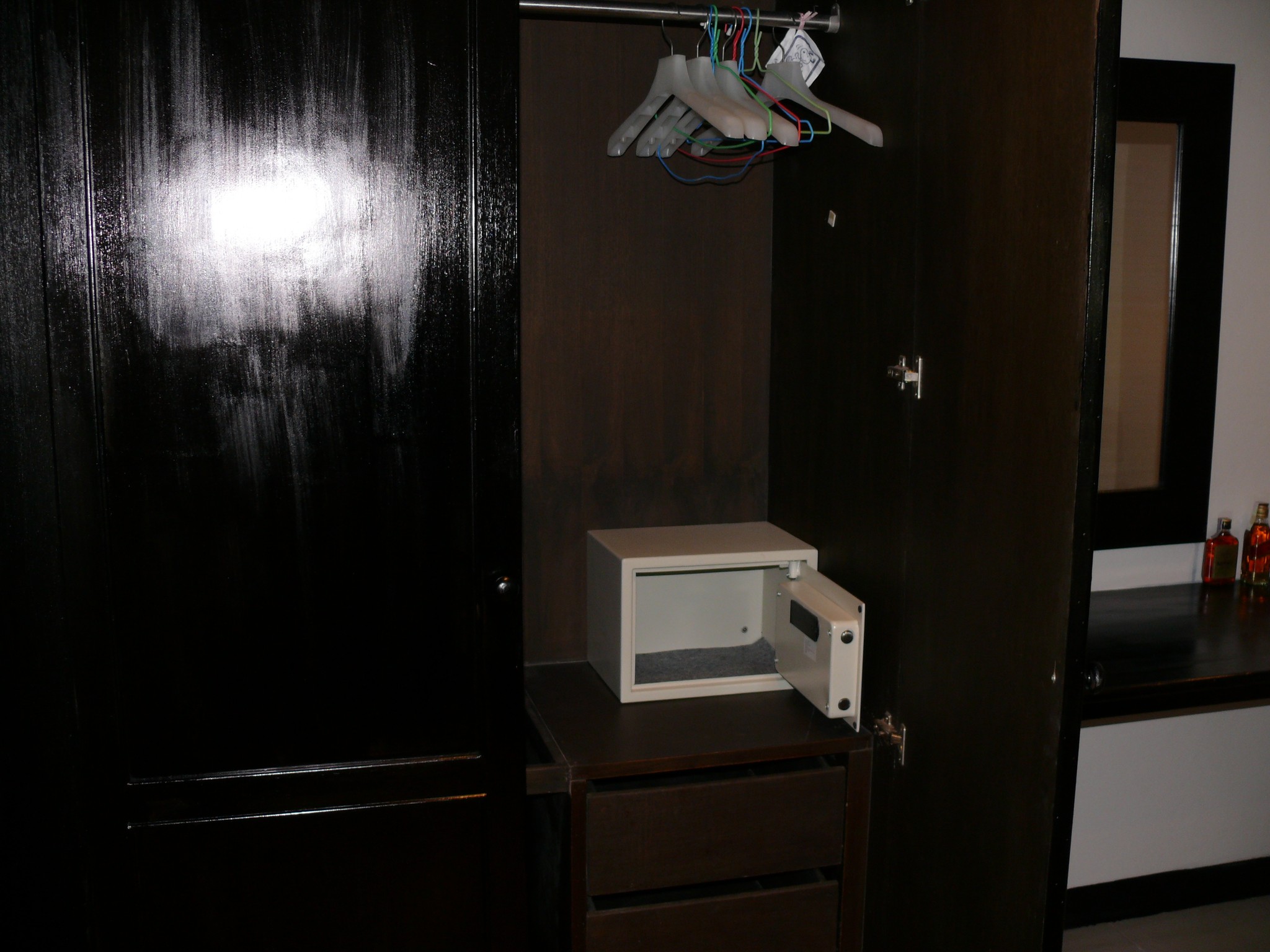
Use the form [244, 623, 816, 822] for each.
[1091, 48, 1244, 552]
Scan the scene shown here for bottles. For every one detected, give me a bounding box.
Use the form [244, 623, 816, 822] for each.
[1240, 504, 1270, 587]
[1201, 517, 1239, 585]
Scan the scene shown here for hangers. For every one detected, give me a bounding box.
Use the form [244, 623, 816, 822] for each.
[607, 4, 888, 188]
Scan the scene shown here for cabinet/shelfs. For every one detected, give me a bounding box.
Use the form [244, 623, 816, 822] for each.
[0, 0, 1125, 952]
[1063, 576, 1270, 932]
